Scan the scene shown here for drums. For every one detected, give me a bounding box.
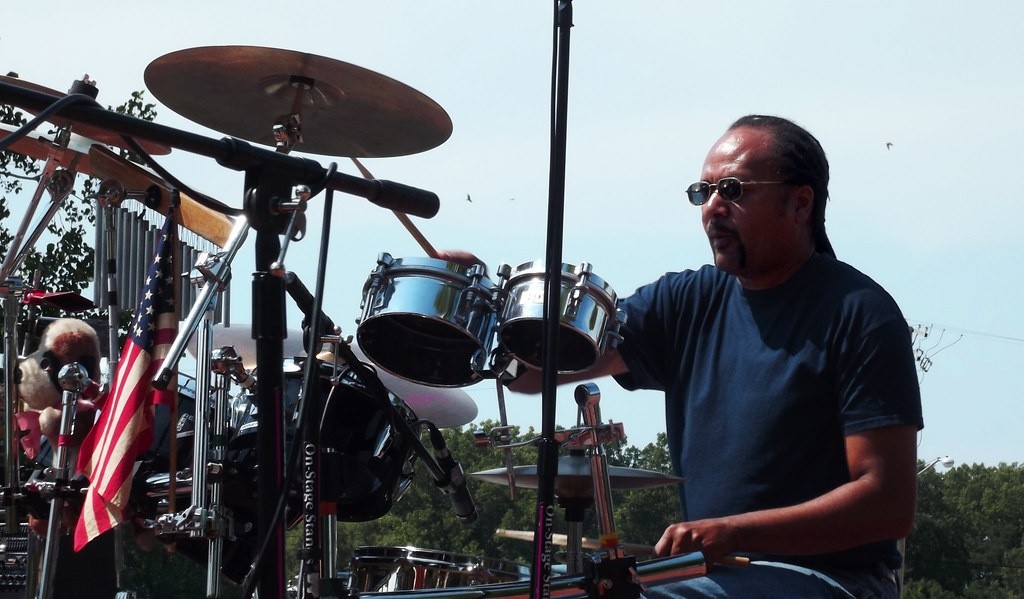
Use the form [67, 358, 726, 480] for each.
[223, 355, 416, 522]
[498, 261, 629, 374]
[345, 545, 584, 599]
[357, 251, 498, 389]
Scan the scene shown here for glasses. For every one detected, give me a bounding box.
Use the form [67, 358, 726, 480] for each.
[686, 177, 806, 205]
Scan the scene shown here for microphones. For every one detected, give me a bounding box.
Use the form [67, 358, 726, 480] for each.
[429, 427, 479, 525]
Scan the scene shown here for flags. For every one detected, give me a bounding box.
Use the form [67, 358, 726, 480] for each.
[73, 208, 181, 554]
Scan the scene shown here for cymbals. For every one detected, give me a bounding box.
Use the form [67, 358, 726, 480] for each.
[1, 72, 172, 180]
[144, 45, 454, 157]
[469, 455, 684, 496]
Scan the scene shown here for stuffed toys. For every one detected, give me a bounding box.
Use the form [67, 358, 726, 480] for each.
[13, 316, 102, 544]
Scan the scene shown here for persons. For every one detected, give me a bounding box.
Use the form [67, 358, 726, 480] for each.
[439, 114, 923, 599]
